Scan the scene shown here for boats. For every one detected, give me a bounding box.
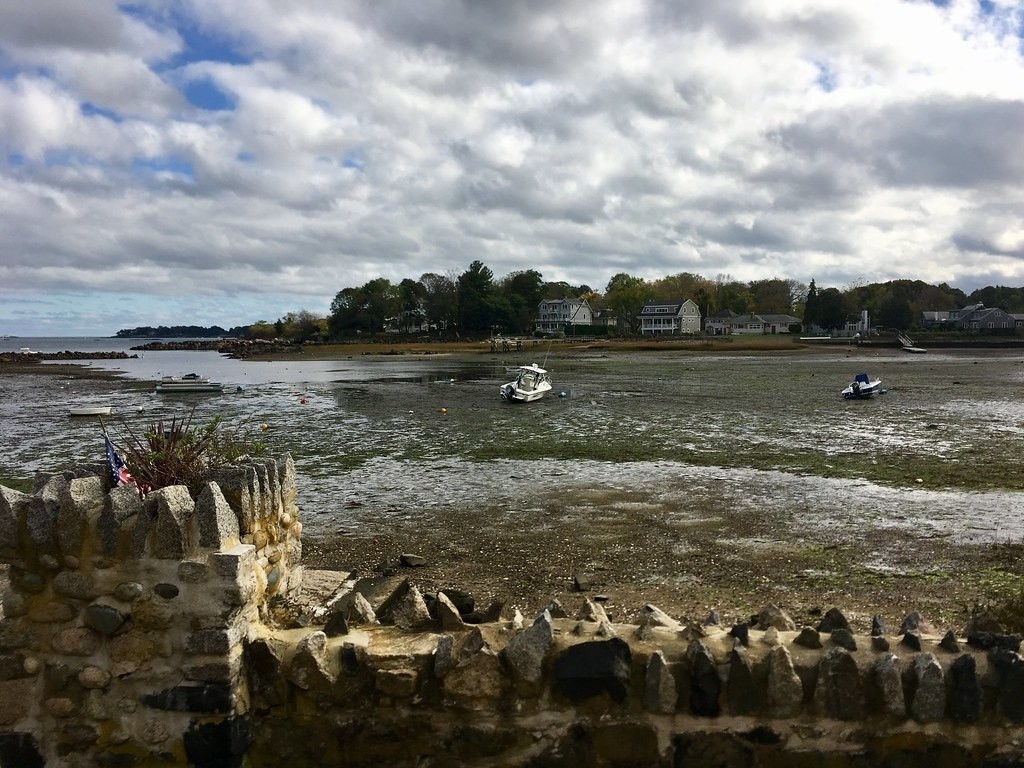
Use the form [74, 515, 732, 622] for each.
[68, 407, 111, 415]
[155, 372, 224, 394]
[841, 374, 882, 400]
[500, 362, 553, 404]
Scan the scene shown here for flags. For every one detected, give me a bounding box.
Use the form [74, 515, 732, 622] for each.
[96, 414, 152, 502]
